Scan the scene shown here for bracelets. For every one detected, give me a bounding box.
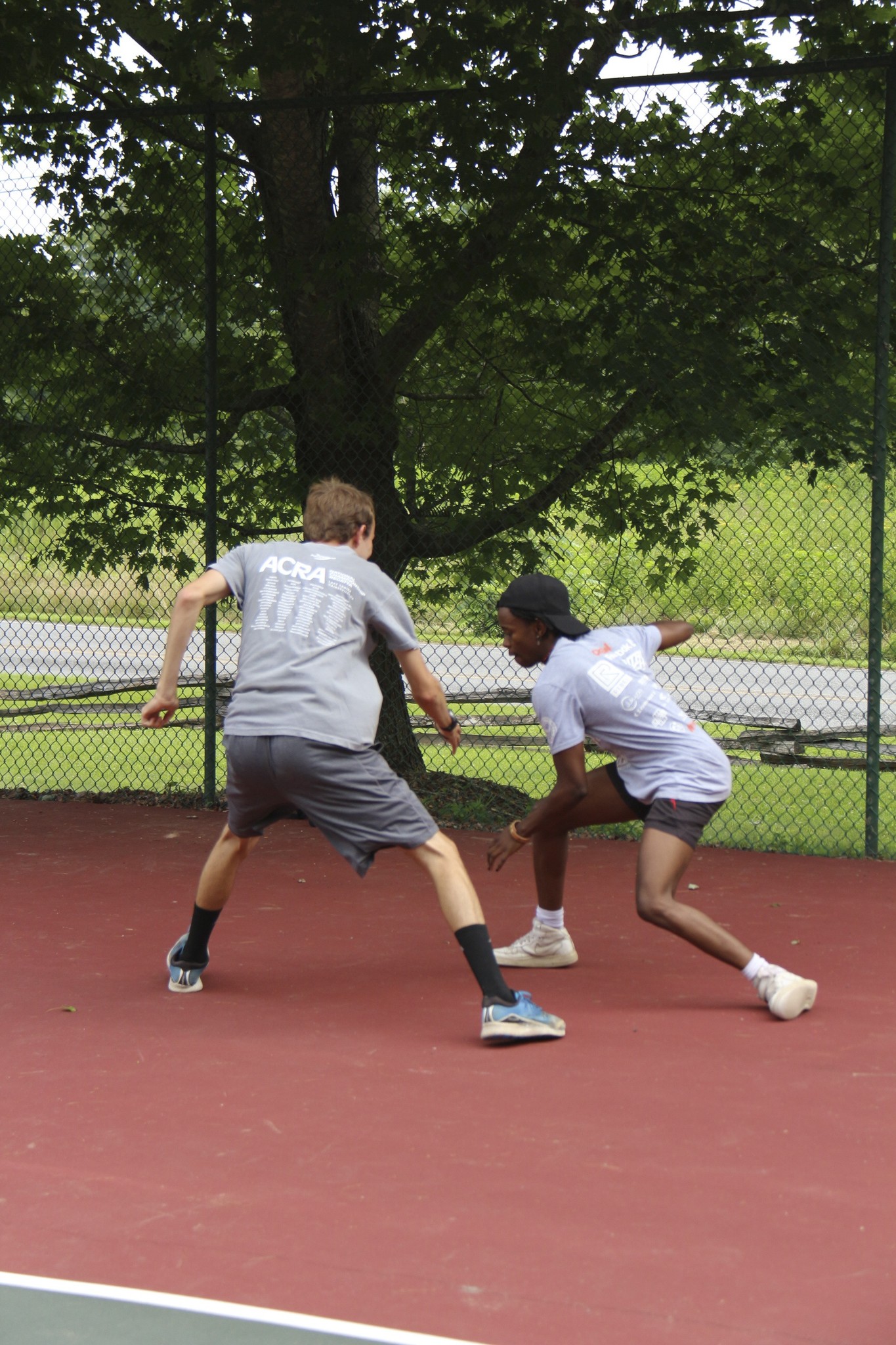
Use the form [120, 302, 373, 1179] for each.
[508, 819, 530, 843]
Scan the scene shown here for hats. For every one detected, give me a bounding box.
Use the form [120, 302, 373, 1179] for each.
[497, 572, 592, 636]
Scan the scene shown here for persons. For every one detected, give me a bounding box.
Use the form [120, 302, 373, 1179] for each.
[485, 574, 818, 1019]
[141, 481, 567, 1042]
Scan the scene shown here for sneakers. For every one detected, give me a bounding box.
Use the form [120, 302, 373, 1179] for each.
[491, 916, 579, 966]
[481, 988, 565, 1043]
[754, 963, 818, 1018]
[165, 931, 209, 994]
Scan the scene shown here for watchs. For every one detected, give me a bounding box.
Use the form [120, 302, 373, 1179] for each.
[439, 708, 457, 732]
